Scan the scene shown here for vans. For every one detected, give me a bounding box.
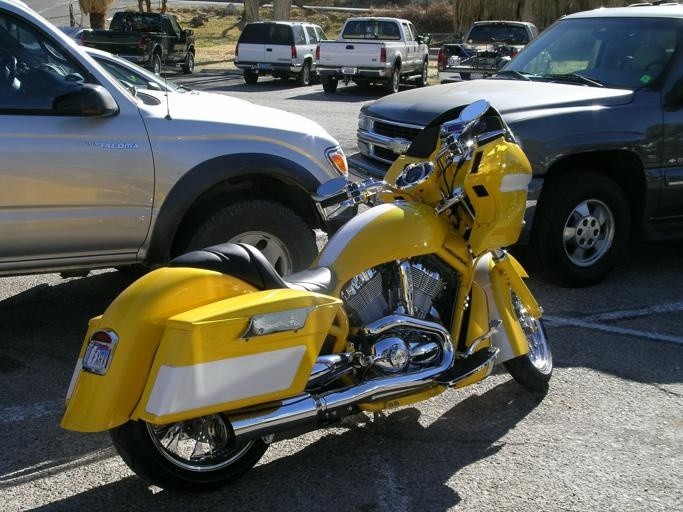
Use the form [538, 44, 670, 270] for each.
[234, 21, 328, 88]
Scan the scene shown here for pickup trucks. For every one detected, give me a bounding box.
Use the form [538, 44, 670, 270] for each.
[82, 10, 196, 76]
[313, 17, 431, 94]
[436, 19, 551, 86]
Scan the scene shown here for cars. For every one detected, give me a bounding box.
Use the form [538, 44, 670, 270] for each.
[55, 25, 110, 46]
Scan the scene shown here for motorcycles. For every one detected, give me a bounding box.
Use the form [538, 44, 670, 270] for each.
[60, 98, 556, 489]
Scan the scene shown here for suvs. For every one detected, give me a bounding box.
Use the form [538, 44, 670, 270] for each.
[0, 0, 352, 280]
[348, 3, 683, 288]
